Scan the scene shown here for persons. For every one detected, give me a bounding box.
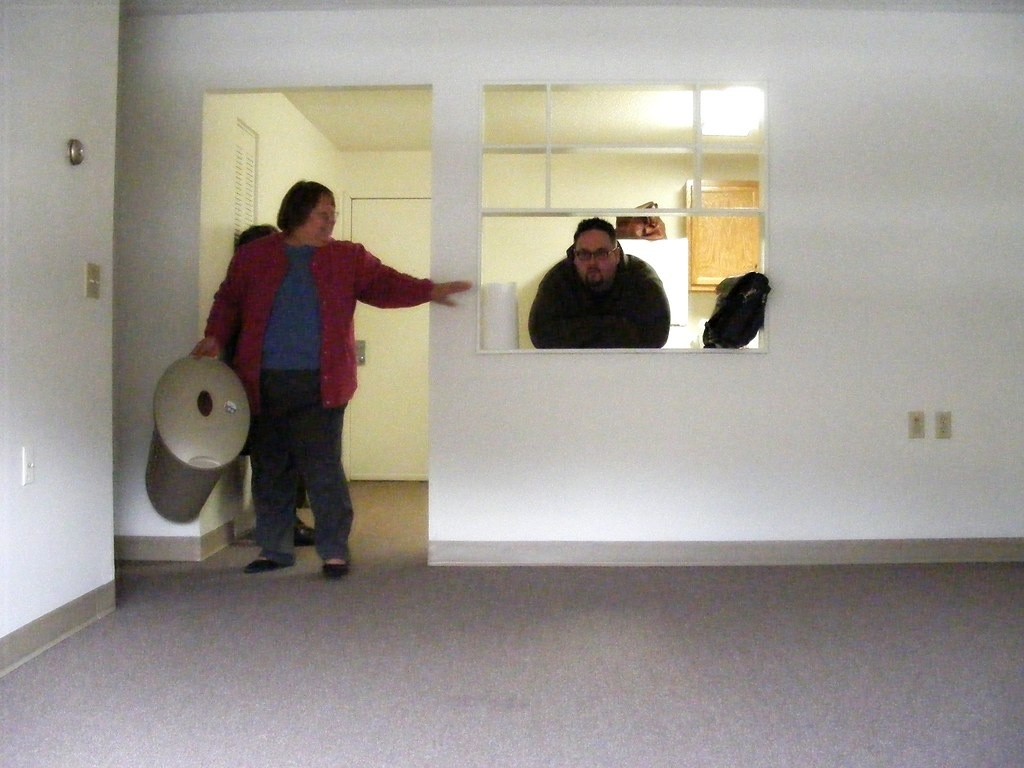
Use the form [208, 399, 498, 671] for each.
[189, 181, 472, 578]
[528, 217, 671, 349]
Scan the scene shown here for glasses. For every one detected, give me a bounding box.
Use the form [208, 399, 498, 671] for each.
[573, 246, 618, 261]
[309, 208, 339, 221]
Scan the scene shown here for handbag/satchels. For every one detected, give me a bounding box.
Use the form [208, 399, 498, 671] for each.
[614, 201, 667, 241]
[703, 272, 771, 348]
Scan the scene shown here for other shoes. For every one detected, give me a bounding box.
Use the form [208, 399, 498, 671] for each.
[322, 560, 348, 576]
[244, 560, 283, 574]
[293, 524, 318, 547]
